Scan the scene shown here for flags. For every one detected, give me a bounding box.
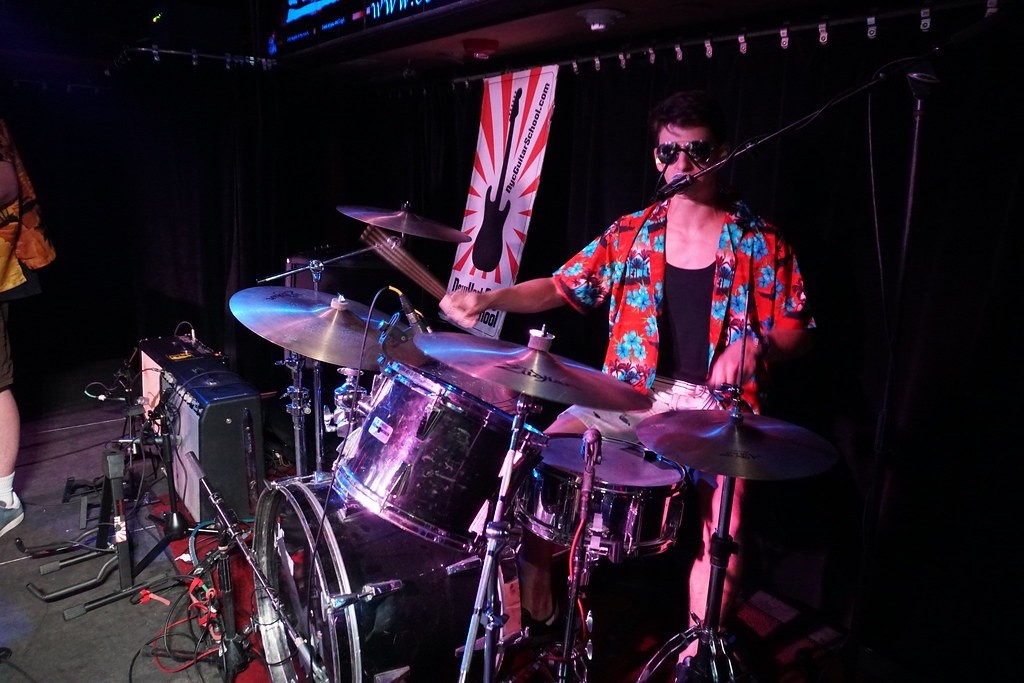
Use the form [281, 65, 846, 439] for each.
[439, 64, 559, 340]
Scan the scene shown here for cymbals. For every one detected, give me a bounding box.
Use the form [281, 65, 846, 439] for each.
[637, 404, 835, 485]
[412, 325, 657, 414]
[227, 280, 433, 373]
[335, 203, 473, 244]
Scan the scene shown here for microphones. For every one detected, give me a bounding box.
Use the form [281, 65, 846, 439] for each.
[389, 285, 425, 335]
[99, 394, 146, 405]
[655, 171, 690, 202]
[581, 429, 601, 493]
[112, 433, 182, 446]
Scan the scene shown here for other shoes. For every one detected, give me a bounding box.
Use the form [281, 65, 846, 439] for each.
[456, 601, 566, 669]
[0, 491, 25, 538]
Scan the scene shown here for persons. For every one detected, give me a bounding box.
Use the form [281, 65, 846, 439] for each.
[0, 120, 56, 538]
[447, 90, 815, 683]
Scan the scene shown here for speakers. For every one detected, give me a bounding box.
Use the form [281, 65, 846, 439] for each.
[284, 256, 432, 369]
[140, 333, 265, 532]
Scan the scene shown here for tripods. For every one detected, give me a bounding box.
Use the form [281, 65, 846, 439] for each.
[140, 387, 331, 682]
[509, 456, 604, 682]
[638, 473, 743, 683]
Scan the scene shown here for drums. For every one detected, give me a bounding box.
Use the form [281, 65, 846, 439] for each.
[512, 430, 687, 558]
[329, 356, 551, 559]
[251, 472, 522, 683]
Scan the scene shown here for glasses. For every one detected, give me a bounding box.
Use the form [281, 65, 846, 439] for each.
[657, 140, 715, 164]
